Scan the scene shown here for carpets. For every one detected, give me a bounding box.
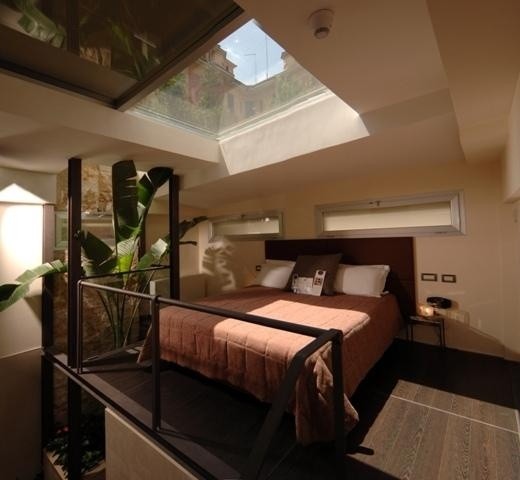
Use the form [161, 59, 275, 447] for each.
[347, 379, 520, 480]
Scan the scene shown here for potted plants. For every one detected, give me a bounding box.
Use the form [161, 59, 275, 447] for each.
[44, 427, 105, 480]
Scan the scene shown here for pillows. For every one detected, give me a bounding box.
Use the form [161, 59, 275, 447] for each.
[285, 253, 344, 296]
[246, 259, 296, 290]
[333, 264, 391, 298]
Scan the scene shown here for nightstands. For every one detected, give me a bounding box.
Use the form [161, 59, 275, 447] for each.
[404, 315, 447, 360]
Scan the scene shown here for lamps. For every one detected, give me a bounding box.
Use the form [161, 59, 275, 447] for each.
[218, 18, 286, 87]
[307, 8, 335, 39]
[427, 296, 451, 316]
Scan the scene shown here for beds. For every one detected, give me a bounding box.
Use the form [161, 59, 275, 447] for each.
[137, 235, 416, 454]
